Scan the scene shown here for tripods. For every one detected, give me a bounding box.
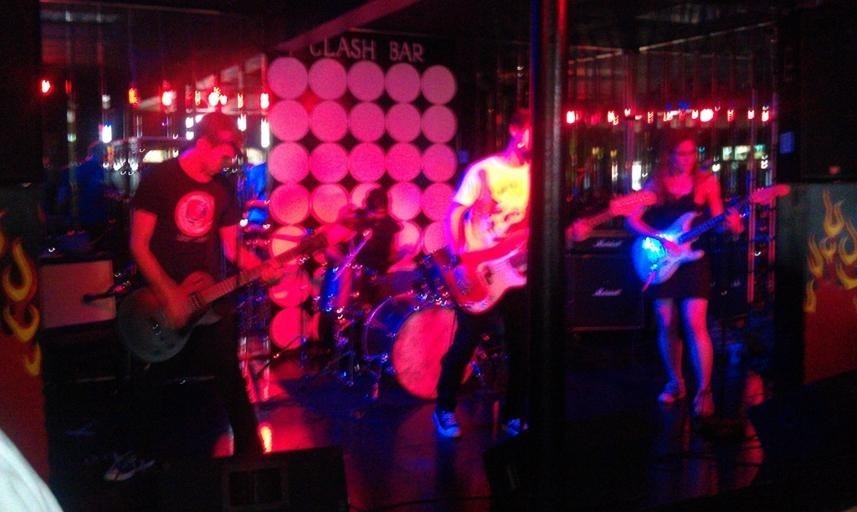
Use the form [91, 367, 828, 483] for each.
[252, 273, 386, 393]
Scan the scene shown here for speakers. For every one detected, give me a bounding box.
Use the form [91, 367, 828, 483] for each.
[565, 229, 645, 329]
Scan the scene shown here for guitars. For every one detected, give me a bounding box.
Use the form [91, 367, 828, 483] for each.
[631, 183, 791, 288]
[116, 233, 328, 363]
[445, 187, 657, 314]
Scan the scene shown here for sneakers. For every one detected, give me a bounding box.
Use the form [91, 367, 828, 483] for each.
[693, 387, 714, 418]
[656, 378, 687, 405]
[432, 409, 461, 438]
[103, 445, 160, 481]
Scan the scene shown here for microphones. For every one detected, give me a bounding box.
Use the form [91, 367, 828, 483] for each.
[83, 290, 113, 305]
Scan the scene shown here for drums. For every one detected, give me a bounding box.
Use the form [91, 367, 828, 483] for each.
[360, 292, 474, 403]
[313, 263, 385, 319]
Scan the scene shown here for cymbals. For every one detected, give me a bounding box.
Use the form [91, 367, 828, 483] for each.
[270, 232, 344, 261]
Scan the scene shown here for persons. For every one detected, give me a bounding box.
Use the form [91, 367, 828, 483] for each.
[430, 104, 533, 442]
[0, 136, 111, 266]
[625, 126, 744, 418]
[100, 112, 285, 483]
[325, 186, 404, 278]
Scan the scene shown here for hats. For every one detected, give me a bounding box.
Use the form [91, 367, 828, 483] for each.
[199, 113, 243, 159]
